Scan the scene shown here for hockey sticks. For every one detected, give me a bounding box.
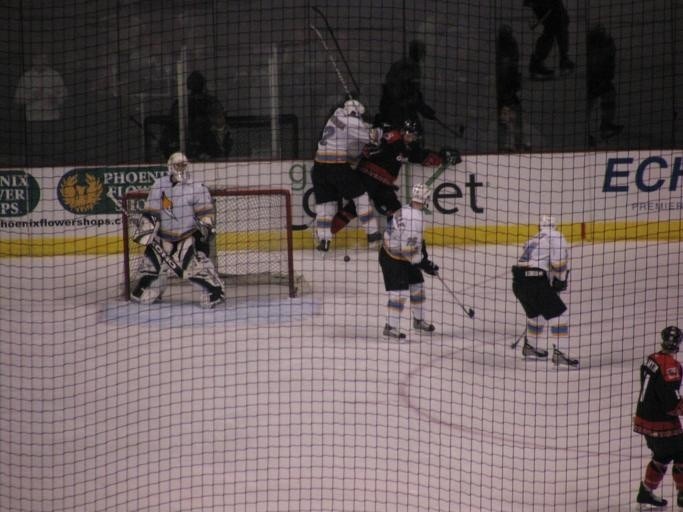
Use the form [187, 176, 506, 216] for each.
[107, 187, 203, 279]
[434, 269, 475, 318]
[511, 328, 526, 348]
[431, 117, 464, 138]
[285, 217, 316, 231]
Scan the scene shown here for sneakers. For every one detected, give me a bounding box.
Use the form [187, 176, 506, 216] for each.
[637, 481, 667, 506]
[522, 336, 578, 366]
[383, 318, 434, 338]
[368, 231, 384, 243]
[317, 240, 330, 251]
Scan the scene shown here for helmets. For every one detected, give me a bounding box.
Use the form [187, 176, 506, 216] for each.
[412, 183, 431, 205]
[661, 326, 682, 353]
[403, 118, 423, 148]
[344, 100, 365, 117]
[168, 153, 190, 183]
[539, 216, 556, 232]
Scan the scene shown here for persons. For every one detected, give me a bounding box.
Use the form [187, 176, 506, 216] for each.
[170, 70, 233, 157]
[378, 35, 438, 144]
[128, 152, 225, 308]
[495, 23, 521, 96]
[12, 47, 67, 165]
[517, 1, 577, 82]
[487, 92, 544, 150]
[378, 183, 439, 339]
[583, 21, 627, 149]
[511, 216, 580, 367]
[633, 327, 682, 507]
[328, 114, 462, 235]
[311, 99, 384, 251]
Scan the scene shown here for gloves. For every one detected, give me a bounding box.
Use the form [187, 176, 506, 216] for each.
[552, 270, 569, 291]
[419, 258, 439, 275]
